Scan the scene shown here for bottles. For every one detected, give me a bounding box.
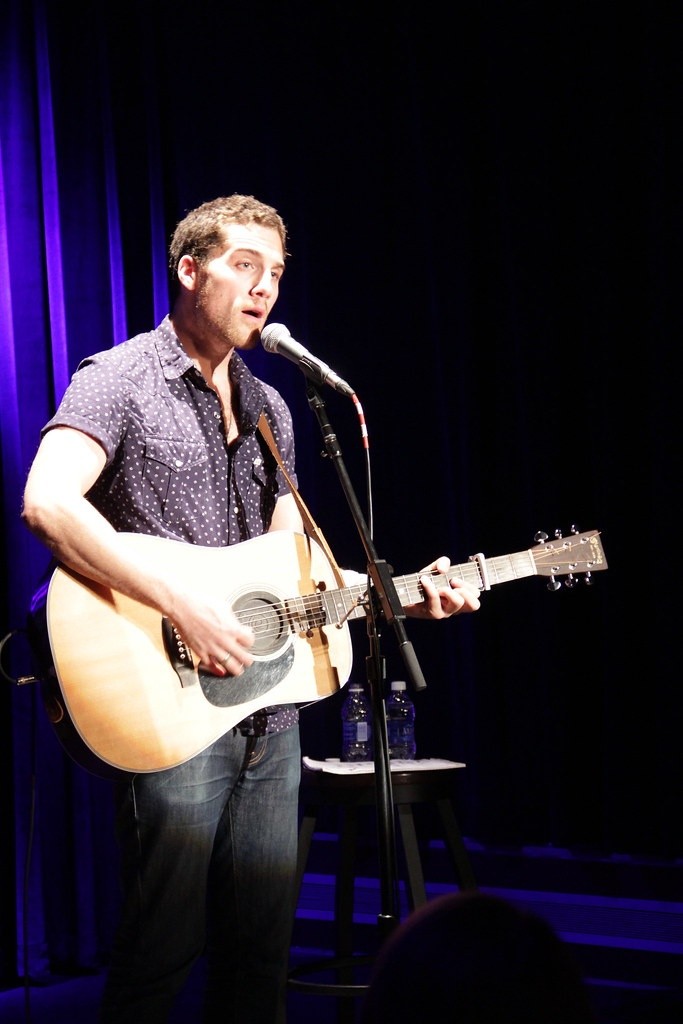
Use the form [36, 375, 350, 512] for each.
[386, 681, 416, 761]
[339, 684, 373, 762]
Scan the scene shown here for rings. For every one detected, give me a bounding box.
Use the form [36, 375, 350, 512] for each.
[221, 652, 231, 666]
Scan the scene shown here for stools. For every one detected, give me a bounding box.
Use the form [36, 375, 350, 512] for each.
[271, 772, 477, 1024]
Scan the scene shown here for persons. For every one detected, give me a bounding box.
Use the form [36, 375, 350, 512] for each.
[23, 195, 481, 1024]
[361, 891, 576, 1024]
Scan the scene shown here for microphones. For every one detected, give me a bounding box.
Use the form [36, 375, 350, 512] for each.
[260, 322, 356, 397]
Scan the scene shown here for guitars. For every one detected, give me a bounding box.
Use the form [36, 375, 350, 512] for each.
[26, 522, 611, 777]
